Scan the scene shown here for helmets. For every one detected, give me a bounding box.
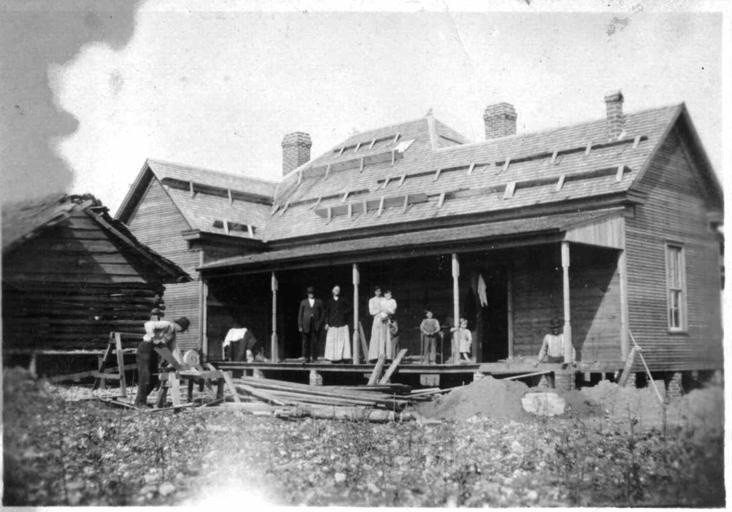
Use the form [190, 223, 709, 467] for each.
[174, 317, 190, 332]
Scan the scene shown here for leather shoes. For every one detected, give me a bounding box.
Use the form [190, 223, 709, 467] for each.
[302, 357, 310, 364]
[158, 401, 173, 408]
[134, 401, 150, 409]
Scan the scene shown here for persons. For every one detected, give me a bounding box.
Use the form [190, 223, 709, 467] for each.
[533, 317, 576, 371]
[298, 287, 325, 362]
[450, 317, 472, 361]
[378, 291, 397, 324]
[420, 309, 441, 364]
[134, 317, 190, 409]
[323, 285, 352, 363]
[368, 286, 398, 363]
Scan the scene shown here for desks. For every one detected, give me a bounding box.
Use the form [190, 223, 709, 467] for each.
[155, 369, 233, 408]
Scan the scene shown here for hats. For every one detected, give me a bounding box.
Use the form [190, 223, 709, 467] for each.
[547, 318, 561, 328]
[305, 287, 316, 295]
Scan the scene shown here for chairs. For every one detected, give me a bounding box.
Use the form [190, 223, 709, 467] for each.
[420, 331, 445, 365]
